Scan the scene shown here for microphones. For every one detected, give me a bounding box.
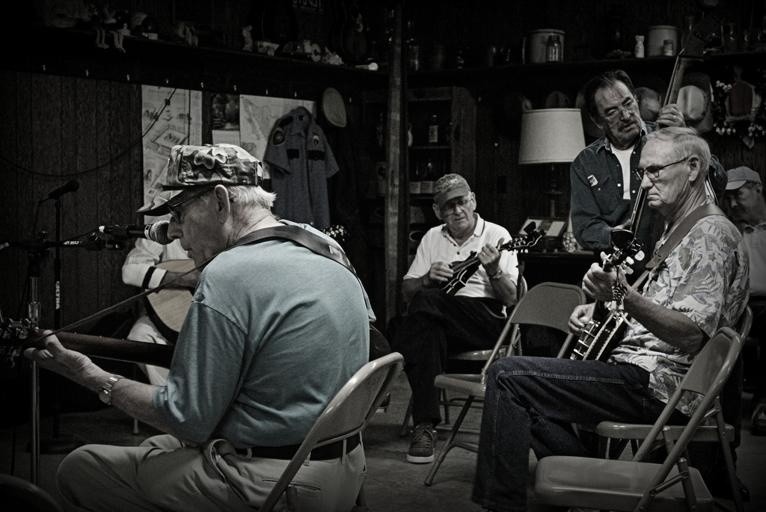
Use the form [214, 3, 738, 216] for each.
[114, 217, 175, 246]
[50, 178, 79, 202]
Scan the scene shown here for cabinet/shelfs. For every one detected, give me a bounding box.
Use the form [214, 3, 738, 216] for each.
[402, 87, 475, 276]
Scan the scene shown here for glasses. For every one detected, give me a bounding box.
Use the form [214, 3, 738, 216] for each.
[634, 158, 688, 182]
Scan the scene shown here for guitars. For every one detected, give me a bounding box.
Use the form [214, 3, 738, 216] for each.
[571, 240, 646, 361]
[439, 233, 534, 294]
[141, 225, 347, 343]
[0, 312, 391, 414]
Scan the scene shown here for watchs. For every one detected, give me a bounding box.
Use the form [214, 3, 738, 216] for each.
[98, 373, 125, 404]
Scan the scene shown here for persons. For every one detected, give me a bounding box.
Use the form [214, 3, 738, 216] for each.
[470, 125, 753, 511]
[398, 173, 521, 460]
[16, 145, 370, 510]
[570, 69, 729, 320]
[722, 164, 766, 435]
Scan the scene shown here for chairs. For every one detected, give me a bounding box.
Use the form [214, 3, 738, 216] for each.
[534, 325, 743, 506]
[439, 274, 530, 426]
[594, 300, 756, 458]
[421, 280, 585, 487]
[256, 348, 406, 512]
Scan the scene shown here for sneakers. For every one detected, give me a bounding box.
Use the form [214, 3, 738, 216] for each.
[406, 421, 438, 464]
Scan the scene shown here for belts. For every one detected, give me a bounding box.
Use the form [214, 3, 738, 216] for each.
[234, 432, 360, 461]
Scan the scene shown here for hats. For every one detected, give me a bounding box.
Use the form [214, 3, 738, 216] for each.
[433, 173, 471, 209]
[723, 166, 763, 192]
[136, 143, 264, 217]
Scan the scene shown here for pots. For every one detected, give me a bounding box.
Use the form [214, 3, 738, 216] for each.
[646, 25, 679, 57]
[521, 29, 568, 64]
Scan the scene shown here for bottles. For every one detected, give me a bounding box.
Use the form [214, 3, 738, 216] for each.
[544, 34, 563, 64]
[411, 111, 445, 194]
[634, 34, 646, 58]
[662, 39, 674, 56]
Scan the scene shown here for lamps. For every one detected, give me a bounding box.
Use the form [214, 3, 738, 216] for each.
[517, 108, 588, 248]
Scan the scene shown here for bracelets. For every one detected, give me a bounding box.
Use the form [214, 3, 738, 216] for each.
[488, 268, 503, 280]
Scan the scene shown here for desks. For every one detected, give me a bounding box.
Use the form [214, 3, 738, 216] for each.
[518, 252, 597, 274]
